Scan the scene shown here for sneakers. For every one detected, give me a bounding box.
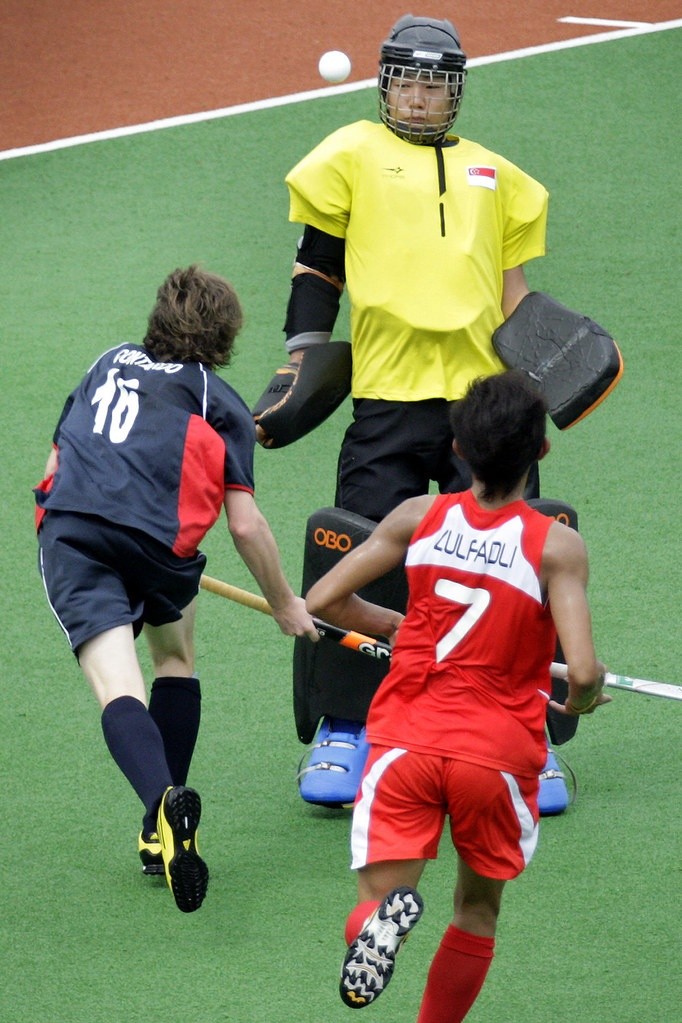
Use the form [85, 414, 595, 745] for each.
[138, 786, 208, 913]
[339, 886, 424, 1008]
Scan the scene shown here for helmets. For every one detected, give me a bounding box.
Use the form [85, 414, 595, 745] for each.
[377, 15, 467, 144]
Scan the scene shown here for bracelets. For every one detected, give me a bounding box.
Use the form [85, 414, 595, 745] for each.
[568, 695, 599, 713]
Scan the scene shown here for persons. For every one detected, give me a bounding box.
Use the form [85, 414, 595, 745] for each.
[33, 267, 322, 919]
[304, 370, 607, 1020]
[251, 13, 626, 818]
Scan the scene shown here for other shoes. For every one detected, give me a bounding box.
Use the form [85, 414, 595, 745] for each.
[537, 728, 569, 814]
[297, 716, 371, 803]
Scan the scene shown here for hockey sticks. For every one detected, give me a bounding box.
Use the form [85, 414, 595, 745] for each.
[181, 566, 394, 663]
[547, 656, 679, 700]
